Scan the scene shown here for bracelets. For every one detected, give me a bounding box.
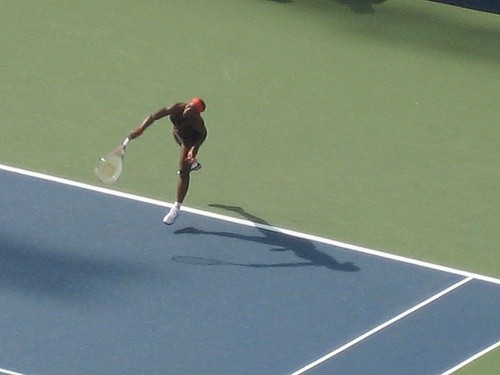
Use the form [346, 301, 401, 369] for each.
[134, 127, 143, 135]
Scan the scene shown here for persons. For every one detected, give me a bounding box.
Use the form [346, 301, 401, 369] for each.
[127, 98, 208, 225]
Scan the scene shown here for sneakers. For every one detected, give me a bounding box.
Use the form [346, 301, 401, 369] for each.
[163, 206, 180, 225]
[176, 161, 201, 175]
